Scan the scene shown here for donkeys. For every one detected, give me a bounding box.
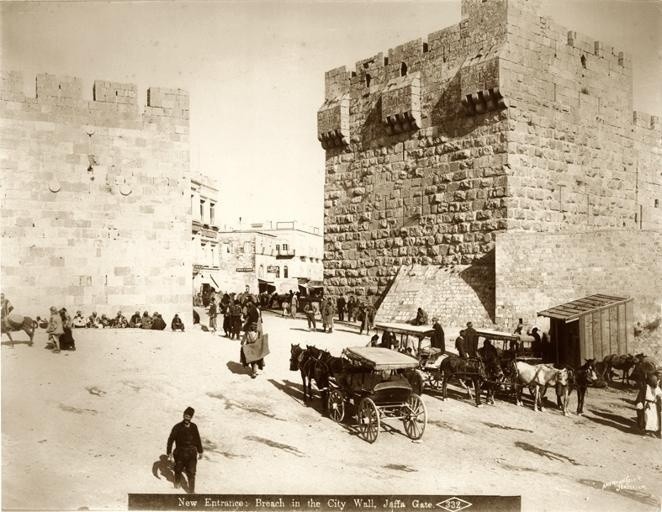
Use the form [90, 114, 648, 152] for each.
[1, 314, 38, 347]
[601, 353, 639, 384]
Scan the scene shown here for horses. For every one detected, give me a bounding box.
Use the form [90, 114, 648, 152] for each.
[439, 353, 597, 417]
[290, 343, 330, 408]
[306, 344, 352, 385]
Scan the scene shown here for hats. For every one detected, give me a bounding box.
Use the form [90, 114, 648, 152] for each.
[183, 407, 195, 417]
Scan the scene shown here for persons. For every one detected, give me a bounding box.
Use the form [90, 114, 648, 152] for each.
[1, 294, 13, 321]
[635, 369, 661, 439]
[36, 305, 186, 352]
[455, 321, 499, 378]
[166, 406, 204, 494]
[431, 318, 445, 350]
[193, 288, 398, 380]
[413, 307, 428, 325]
